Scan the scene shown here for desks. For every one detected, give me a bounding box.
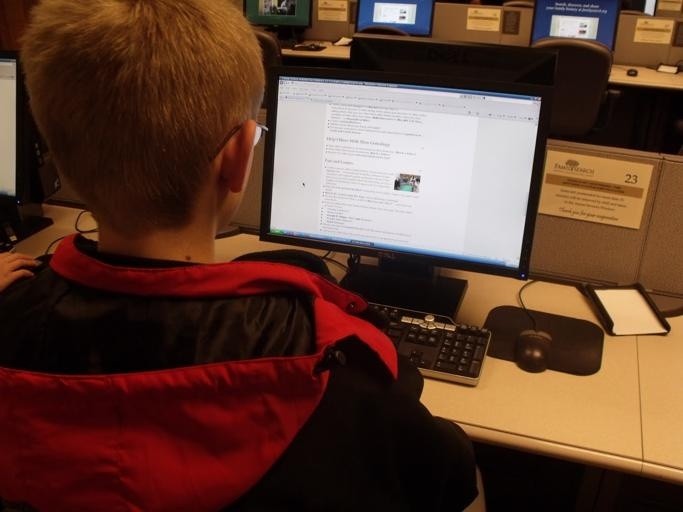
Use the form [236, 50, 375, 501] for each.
[283, 36, 681, 88]
[1, 198, 683, 486]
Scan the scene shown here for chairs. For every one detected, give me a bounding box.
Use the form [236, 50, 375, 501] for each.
[535, 36, 612, 138]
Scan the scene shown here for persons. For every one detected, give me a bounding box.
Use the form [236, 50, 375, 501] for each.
[271, 1, 291, 15]
[1, 1, 481, 512]
[395, 174, 420, 193]
[1, 251, 41, 291]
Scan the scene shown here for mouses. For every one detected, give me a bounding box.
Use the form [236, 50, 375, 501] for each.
[627, 69, 637, 76]
[517, 328, 552, 373]
[309, 43, 319, 50]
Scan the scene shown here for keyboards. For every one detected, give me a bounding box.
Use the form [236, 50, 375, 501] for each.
[362, 301, 493, 386]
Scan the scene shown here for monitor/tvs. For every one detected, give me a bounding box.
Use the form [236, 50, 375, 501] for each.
[529, 0, 621, 53]
[258, 65, 551, 321]
[243, 0, 313, 49]
[0, 48, 54, 252]
[354, 0, 435, 37]
